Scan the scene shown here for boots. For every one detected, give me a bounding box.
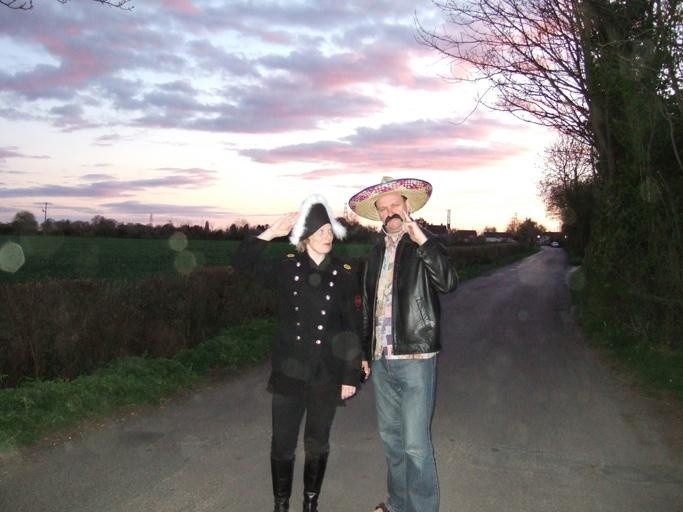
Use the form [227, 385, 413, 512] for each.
[302, 454, 328, 512]
[269, 455, 296, 512]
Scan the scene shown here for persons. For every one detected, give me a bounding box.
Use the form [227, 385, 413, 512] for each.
[230, 200, 361, 511]
[347, 175, 457, 511]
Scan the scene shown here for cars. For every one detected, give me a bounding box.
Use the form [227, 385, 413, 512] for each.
[550, 241, 559, 248]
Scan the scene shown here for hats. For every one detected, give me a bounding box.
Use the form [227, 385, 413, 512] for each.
[347, 173, 434, 222]
[295, 203, 331, 244]
